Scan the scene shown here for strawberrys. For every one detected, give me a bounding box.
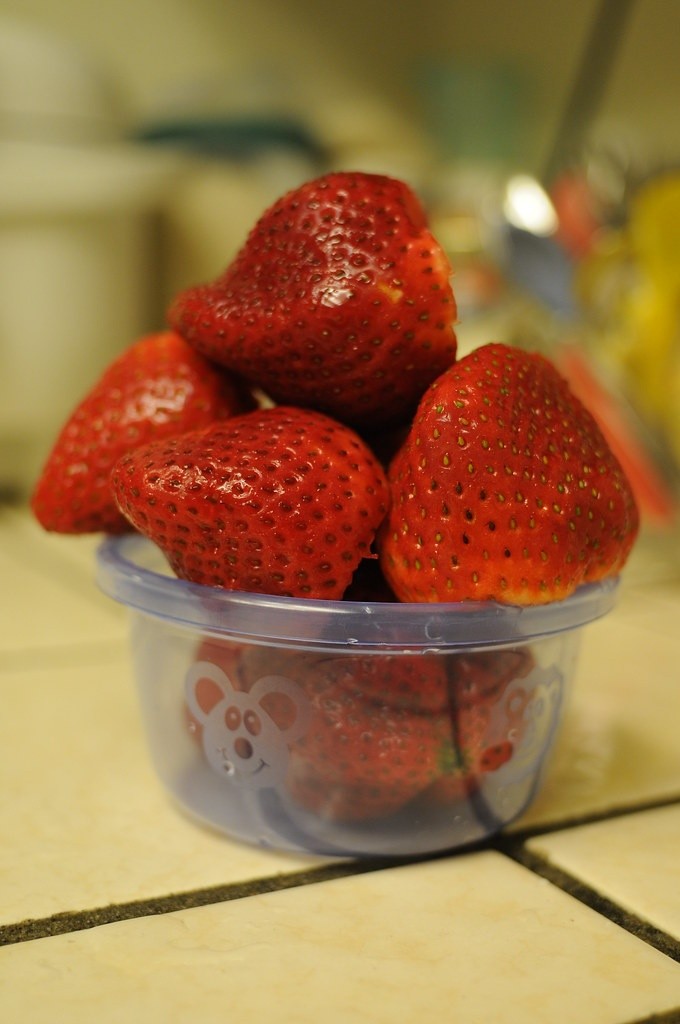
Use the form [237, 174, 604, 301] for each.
[32, 169, 640, 826]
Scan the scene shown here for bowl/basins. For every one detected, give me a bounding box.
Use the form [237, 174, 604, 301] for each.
[99, 536, 616, 857]
